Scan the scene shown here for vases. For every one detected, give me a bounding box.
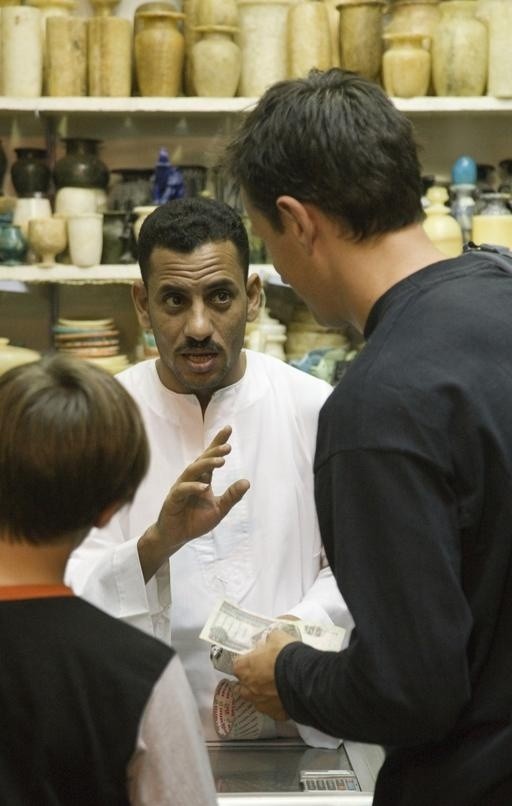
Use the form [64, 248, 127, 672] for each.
[1, 0, 512, 267]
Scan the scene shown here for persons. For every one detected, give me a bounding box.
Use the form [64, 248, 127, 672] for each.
[64, 196, 356, 755]
[227, 66, 512, 804]
[2, 353, 220, 804]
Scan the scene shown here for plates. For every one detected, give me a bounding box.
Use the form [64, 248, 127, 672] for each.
[51, 315, 133, 371]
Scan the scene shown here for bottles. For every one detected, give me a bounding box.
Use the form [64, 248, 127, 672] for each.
[1, 0, 512, 105]
[1, 111, 512, 268]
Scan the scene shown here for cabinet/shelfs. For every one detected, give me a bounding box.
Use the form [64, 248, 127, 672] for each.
[1, 96, 512, 282]
